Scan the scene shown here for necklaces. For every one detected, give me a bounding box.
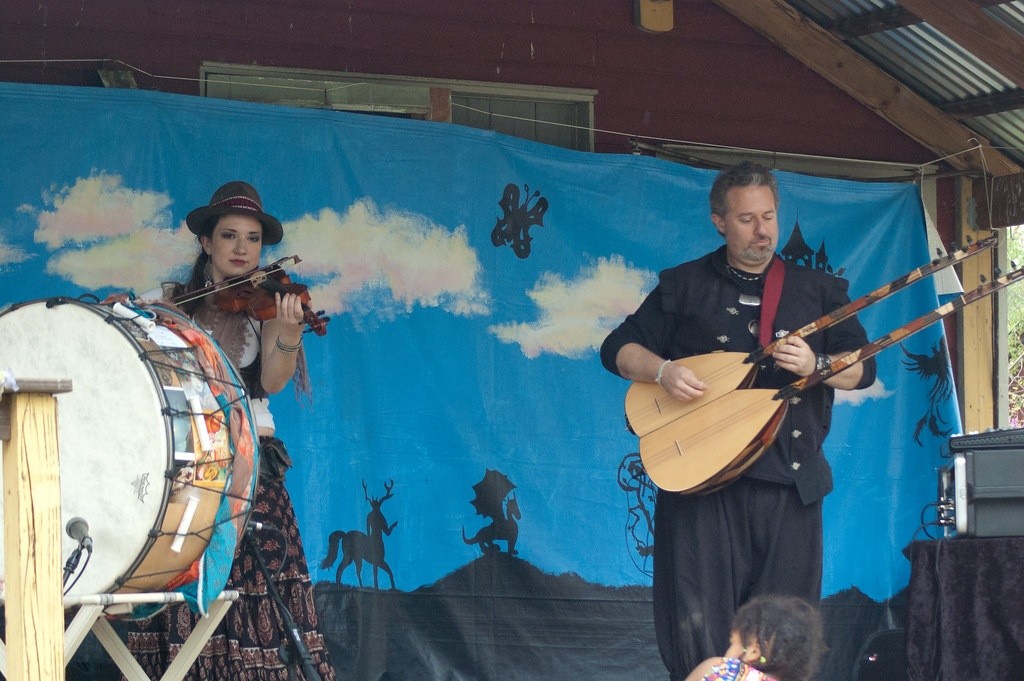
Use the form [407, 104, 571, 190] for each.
[727, 265, 761, 281]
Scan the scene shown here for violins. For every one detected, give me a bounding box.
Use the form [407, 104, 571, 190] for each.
[214, 263, 332, 338]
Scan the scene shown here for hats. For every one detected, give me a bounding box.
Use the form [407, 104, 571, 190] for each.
[185, 181, 283, 246]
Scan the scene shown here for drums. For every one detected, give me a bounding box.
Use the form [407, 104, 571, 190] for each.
[0, 288, 261, 611]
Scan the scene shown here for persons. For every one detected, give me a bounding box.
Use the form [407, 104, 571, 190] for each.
[120, 181, 339, 681]
[600, 162, 877, 681]
[684, 594, 826, 681]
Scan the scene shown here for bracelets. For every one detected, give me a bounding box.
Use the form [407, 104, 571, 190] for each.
[655, 360, 670, 385]
[816, 354, 832, 371]
[276, 336, 302, 354]
